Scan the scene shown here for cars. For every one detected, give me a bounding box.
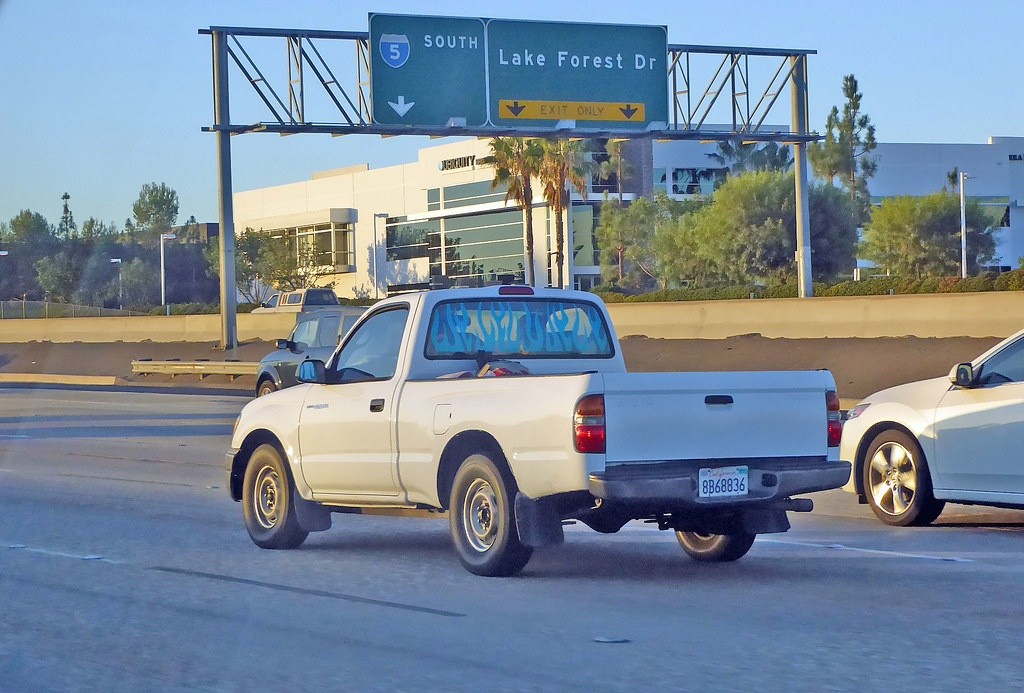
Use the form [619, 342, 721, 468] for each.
[253, 305, 370, 400]
[836, 328, 1024, 526]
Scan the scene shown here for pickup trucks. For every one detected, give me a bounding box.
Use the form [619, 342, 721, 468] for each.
[224, 279, 853, 579]
[249, 287, 341, 314]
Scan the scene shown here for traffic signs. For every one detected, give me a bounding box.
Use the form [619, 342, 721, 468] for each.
[484, 17, 669, 131]
[368, 11, 491, 128]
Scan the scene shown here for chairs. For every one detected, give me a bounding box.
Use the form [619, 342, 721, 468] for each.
[517, 312, 545, 338]
[428, 332, 485, 353]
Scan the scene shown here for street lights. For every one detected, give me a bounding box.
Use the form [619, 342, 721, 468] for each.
[373, 212, 388, 299]
[160, 233, 177, 305]
[110, 258, 123, 310]
